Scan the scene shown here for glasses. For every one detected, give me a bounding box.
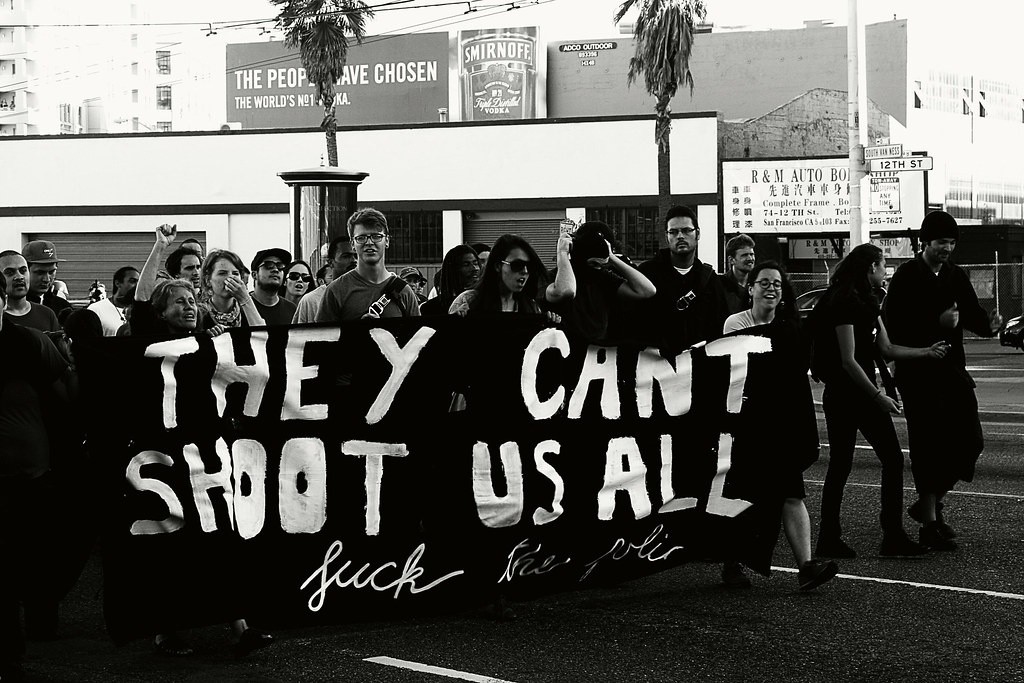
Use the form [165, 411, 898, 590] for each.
[667, 226, 696, 235]
[285, 272, 311, 282]
[256, 260, 286, 272]
[353, 233, 387, 245]
[501, 258, 534, 274]
[750, 279, 782, 290]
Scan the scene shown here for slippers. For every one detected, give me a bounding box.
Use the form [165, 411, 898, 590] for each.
[154, 636, 193, 657]
[235, 629, 275, 658]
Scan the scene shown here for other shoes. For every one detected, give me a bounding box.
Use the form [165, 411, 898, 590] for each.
[479, 594, 516, 621]
[879, 535, 929, 558]
[816, 538, 857, 561]
[797, 559, 840, 590]
[722, 563, 753, 594]
[919, 527, 958, 550]
[906, 501, 955, 537]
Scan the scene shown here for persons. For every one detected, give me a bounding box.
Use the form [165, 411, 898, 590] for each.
[634, 205, 728, 342]
[144, 279, 272, 656]
[872, 210, 1004, 552]
[0, 239, 102, 683]
[715, 262, 839, 589]
[724, 232, 756, 284]
[544, 222, 656, 310]
[90, 224, 318, 337]
[804, 243, 952, 559]
[292, 207, 428, 325]
[419, 234, 563, 323]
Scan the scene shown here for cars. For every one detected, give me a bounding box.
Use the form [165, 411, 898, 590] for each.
[795, 274, 892, 327]
[998, 313, 1024, 352]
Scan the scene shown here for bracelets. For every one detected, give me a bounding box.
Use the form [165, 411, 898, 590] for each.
[873, 390, 881, 401]
[238, 297, 252, 306]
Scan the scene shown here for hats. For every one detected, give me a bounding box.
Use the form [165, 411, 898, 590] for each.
[399, 267, 419, 279]
[22, 240, 65, 263]
[570, 220, 614, 260]
[250, 247, 292, 272]
